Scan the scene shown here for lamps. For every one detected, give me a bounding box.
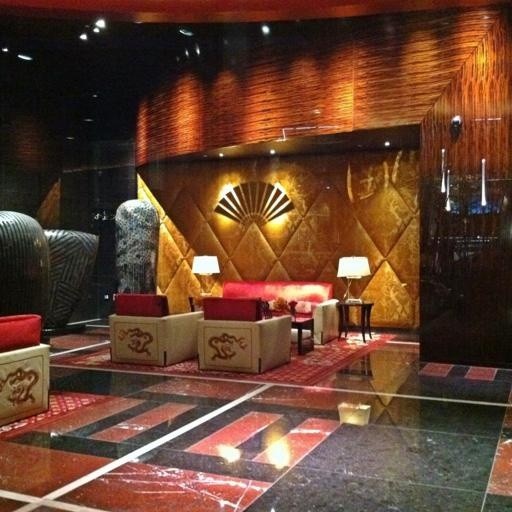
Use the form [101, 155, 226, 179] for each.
[336, 256, 372, 305]
[191, 255, 220, 296]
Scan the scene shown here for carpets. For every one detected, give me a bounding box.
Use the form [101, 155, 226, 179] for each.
[0, 390, 118, 437]
[50, 331, 398, 386]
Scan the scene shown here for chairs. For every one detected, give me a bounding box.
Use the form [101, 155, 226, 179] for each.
[109, 294, 291, 374]
[0, 313, 51, 428]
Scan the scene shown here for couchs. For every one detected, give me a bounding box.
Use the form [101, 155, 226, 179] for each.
[189, 280, 340, 345]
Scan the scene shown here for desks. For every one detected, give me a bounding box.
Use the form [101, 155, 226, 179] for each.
[336, 303, 374, 344]
